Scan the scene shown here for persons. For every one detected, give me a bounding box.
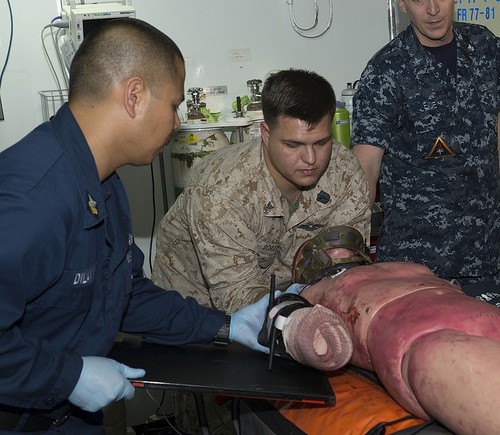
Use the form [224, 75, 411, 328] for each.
[353, 0, 499, 309]
[150, 68, 371, 435]
[258, 225, 500, 435]
[0, 18, 289, 435]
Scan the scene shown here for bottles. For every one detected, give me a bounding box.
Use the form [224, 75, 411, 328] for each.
[342, 83, 358, 135]
[332, 101, 351, 147]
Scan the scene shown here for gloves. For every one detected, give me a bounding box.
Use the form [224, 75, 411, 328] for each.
[229, 289, 282, 354]
[67, 355, 146, 413]
